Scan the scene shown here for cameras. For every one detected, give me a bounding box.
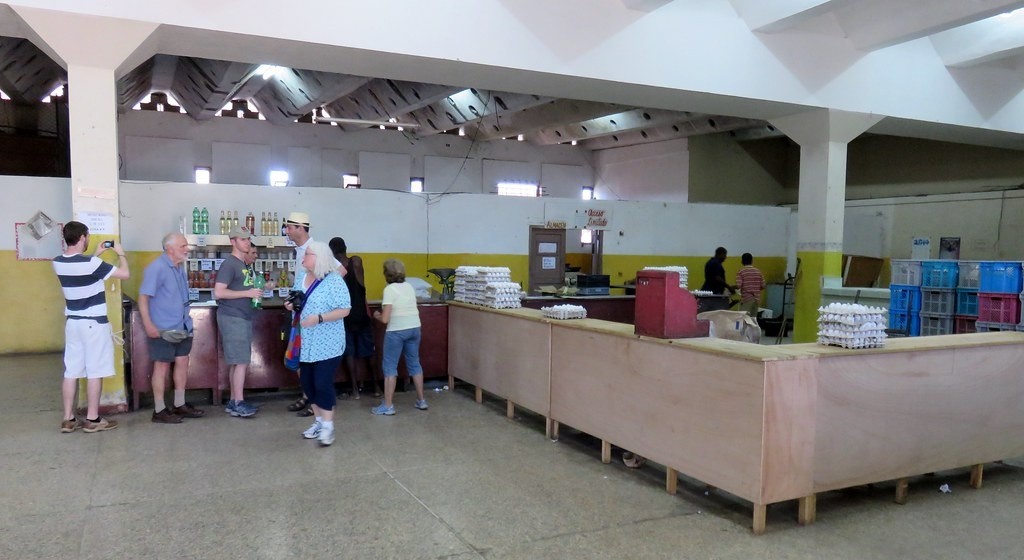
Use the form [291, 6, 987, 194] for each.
[105, 241, 114, 248]
[288, 290, 306, 312]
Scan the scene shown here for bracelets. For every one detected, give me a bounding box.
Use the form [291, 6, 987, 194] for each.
[117, 254, 127, 258]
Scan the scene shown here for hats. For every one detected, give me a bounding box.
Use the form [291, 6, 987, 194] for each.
[229, 226, 254, 239]
[282, 213, 313, 228]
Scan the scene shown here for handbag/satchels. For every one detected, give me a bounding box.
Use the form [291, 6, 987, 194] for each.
[285, 313, 301, 371]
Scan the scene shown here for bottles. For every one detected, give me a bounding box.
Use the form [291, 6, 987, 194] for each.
[192, 205, 200, 234]
[246, 212, 255, 236]
[282, 217, 287, 236]
[201, 207, 209, 234]
[260, 212, 279, 236]
[250, 270, 265, 309]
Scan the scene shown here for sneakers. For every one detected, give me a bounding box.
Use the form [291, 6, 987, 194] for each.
[231, 401, 256, 417]
[82, 417, 118, 433]
[415, 399, 428, 408]
[318, 425, 335, 446]
[225, 400, 259, 412]
[61, 417, 85, 432]
[300, 421, 322, 439]
[370, 399, 396, 415]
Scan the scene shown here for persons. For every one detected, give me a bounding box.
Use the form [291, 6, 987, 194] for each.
[700, 246, 736, 295]
[53, 221, 131, 432]
[283, 241, 351, 446]
[735, 253, 766, 317]
[213, 226, 276, 415]
[137, 231, 206, 423]
[283, 212, 384, 417]
[371, 259, 428, 415]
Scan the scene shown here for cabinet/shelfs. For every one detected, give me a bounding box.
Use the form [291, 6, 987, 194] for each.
[180, 216, 298, 302]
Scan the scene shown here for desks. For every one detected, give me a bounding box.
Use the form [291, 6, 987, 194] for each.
[445, 300, 1024, 534]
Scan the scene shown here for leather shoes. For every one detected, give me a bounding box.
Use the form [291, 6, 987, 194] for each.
[151, 406, 182, 423]
[172, 402, 205, 417]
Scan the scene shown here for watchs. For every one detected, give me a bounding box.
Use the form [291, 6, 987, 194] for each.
[318, 314, 323, 323]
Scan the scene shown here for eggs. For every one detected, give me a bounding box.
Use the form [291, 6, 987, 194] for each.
[643, 265, 688, 288]
[552, 304, 584, 312]
[821, 302, 883, 345]
[452, 265, 521, 307]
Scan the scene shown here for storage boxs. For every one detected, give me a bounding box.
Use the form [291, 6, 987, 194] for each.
[577, 275, 610, 295]
[889, 259, 1024, 337]
[765, 321, 789, 337]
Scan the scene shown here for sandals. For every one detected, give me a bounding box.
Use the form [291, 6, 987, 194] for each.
[287, 397, 308, 412]
[297, 406, 315, 417]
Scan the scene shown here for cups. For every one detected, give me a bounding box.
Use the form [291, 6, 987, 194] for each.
[219, 210, 240, 235]
